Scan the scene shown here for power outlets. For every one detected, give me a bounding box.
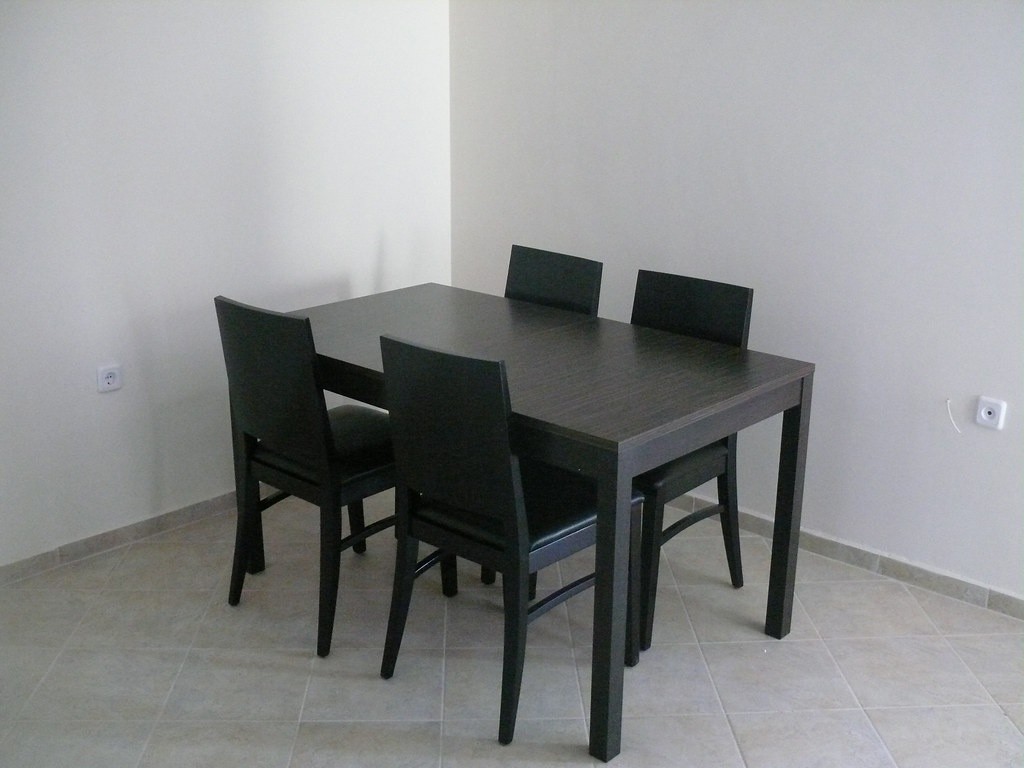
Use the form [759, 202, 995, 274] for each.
[974, 396, 1007, 430]
[96, 363, 123, 393]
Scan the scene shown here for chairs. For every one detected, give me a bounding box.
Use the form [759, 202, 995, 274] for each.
[480, 244, 604, 586]
[518, 269, 754, 651]
[213, 295, 458, 658]
[380, 335, 647, 746]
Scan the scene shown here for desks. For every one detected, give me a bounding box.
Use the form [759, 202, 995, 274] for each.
[230, 283, 817, 763]
